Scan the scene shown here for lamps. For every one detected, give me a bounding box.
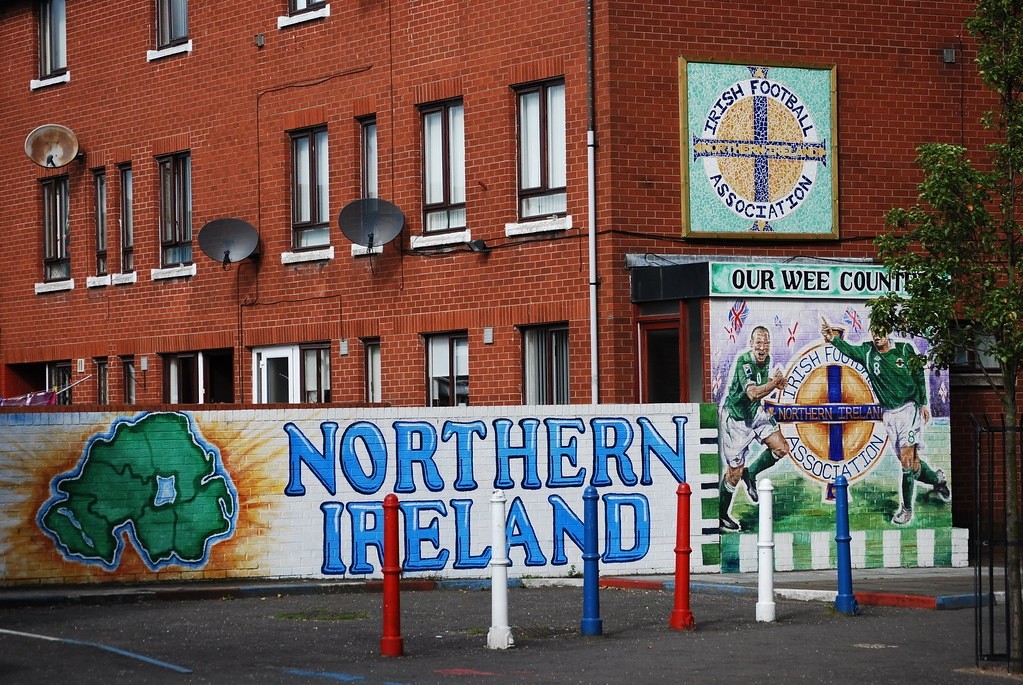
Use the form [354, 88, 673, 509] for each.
[467, 239, 485, 256]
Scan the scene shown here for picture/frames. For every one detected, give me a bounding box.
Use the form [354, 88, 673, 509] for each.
[678, 57, 838, 242]
